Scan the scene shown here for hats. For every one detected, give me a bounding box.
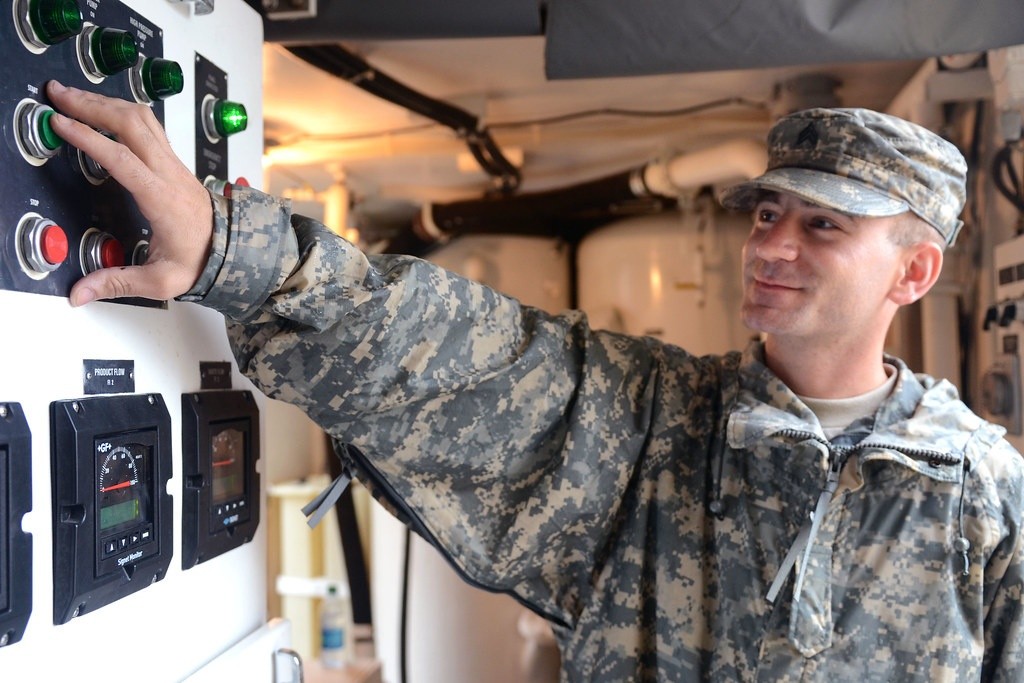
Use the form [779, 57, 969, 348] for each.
[718, 108, 969, 247]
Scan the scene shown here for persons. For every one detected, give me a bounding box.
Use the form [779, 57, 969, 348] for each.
[49, 80, 1024, 683]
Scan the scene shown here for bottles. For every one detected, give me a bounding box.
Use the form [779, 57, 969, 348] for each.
[320, 587, 345, 669]
